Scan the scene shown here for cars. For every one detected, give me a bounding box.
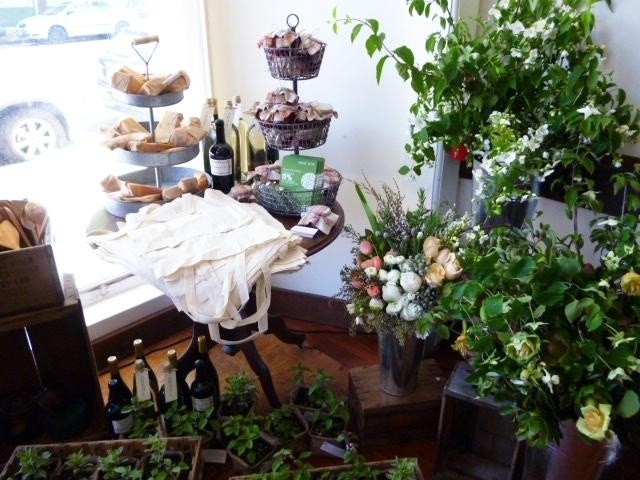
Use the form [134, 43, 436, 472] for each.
[17, 0, 140, 43]
[0, 58, 103, 165]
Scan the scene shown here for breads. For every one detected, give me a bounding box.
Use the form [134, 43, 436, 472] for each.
[110, 67, 191, 96]
[100, 172, 208, 202]
[95, 111, 209, 153]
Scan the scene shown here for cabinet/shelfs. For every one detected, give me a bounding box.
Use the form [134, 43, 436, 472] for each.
[1, 273, 113, 443]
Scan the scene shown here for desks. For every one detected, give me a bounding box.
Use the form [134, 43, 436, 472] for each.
[83, 191, 344, 410]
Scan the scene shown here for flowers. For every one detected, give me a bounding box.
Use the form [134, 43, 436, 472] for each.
[327, 0, 639, 214]
[326, 180, 488, 349]
[441, 210, 640, 447]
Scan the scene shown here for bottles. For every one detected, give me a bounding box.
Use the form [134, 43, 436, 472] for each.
[201, 98, 219, 176]
[189, 359, 216, 420]
[158, 363, 193, 415]
[245, 101, 270, 172]
[106, 378, 136, 439]
[167, 349, 192, 396]
[132, 338, 160, 398]
[130, 358, 162, 422]
[209, 120, 234, 195]
[107, 355, 133, 405]
[222, 99, 242, 186]
[234, 95, 248, 173]
[194, 334, 222, 408]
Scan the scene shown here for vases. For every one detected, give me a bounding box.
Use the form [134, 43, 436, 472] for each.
[472, 159, 529, 230]
[374, 325, 425, 397]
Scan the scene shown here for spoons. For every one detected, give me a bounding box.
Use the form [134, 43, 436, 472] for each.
[19, 208, 39, 245]
[23, 202, 46, 237]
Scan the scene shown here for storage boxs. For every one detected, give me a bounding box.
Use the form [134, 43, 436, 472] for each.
[0, 218, 65, 313]
[349, 359, 446, 452]
